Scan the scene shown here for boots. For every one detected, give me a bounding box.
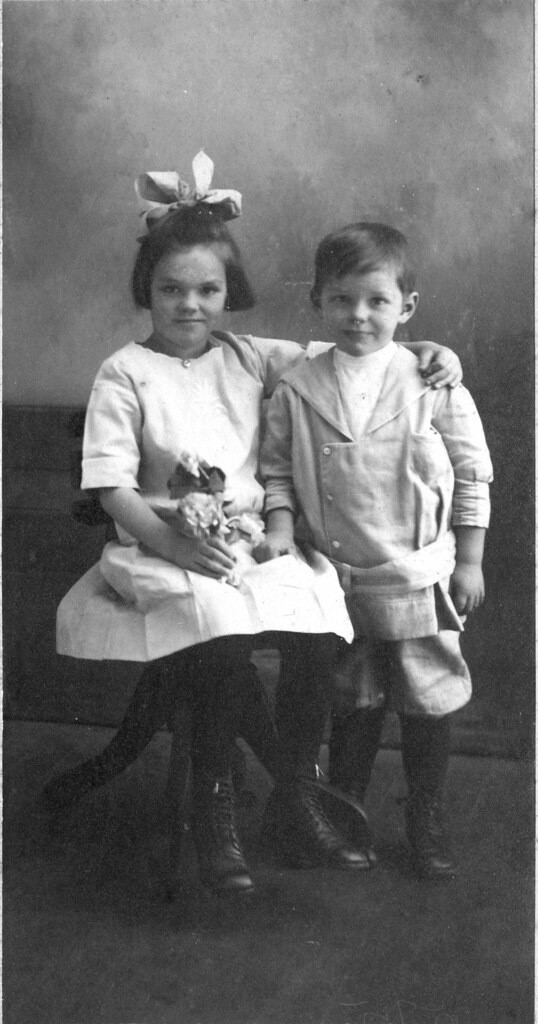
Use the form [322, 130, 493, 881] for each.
[189, 757, 255, 897]
[330, 702, 386, 870]
[395, 710, 457, 879]
[253, 755, 376, 871]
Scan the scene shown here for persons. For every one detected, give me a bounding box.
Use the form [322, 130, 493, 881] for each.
[57, 148, 464, 896]
[254, 222, 493, 876]
